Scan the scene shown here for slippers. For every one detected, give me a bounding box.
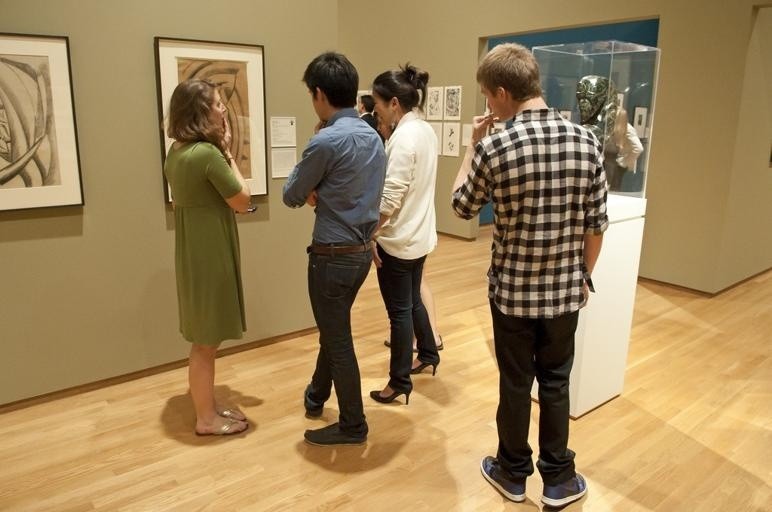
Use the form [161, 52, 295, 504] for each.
[195, 419, 249, 436]
[218, 406, 248, 423]
[383, 341, 391, 347]
[436, 335, 443, 350]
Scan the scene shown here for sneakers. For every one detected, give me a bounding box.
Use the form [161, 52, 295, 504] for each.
[540, 473, 588, 509]
[304, 423, 369, 447]
[480, 455, 527, 504]
[303, 385, 324, 420]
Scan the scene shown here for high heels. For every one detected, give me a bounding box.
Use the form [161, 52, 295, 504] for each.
[410, 352, 440, 376]
[369, 381, 413, 405]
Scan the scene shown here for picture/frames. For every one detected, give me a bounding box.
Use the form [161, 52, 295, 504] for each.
[0, 33, 86, 211]
[154, 36, 269, 203]
[633, 105, 648, 139]
[558, 108, 572, 121]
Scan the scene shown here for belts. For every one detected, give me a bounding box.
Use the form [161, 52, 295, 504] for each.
[311, 239, 373, 256]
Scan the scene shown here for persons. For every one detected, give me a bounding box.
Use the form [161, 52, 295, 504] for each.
[281, 51, 382, 447]
[610, 122, 645, 191]
[377, 114, 445, 353]
[366, 58, 440, 408]
[163, 81, 251, 435]
[451, 42, 608, 506]
[356, 94, 377, 130]
